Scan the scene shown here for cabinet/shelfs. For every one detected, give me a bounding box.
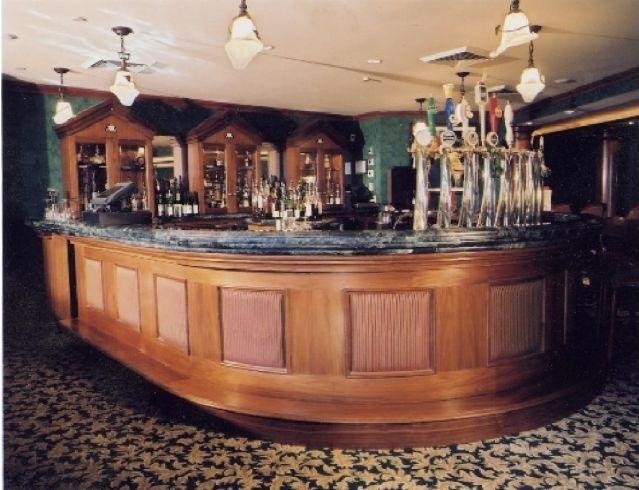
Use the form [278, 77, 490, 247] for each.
[53, 99, 355, 222]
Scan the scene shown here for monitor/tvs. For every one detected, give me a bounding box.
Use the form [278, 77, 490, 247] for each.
[92, 182, 134, 205]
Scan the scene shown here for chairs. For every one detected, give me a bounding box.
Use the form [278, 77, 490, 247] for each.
[555, 204, 639, 370]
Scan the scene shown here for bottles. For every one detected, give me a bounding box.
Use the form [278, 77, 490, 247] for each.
[242, 174, 324, 221]
[76, 143, 105, 167]
[152, 173, 200, 220]
